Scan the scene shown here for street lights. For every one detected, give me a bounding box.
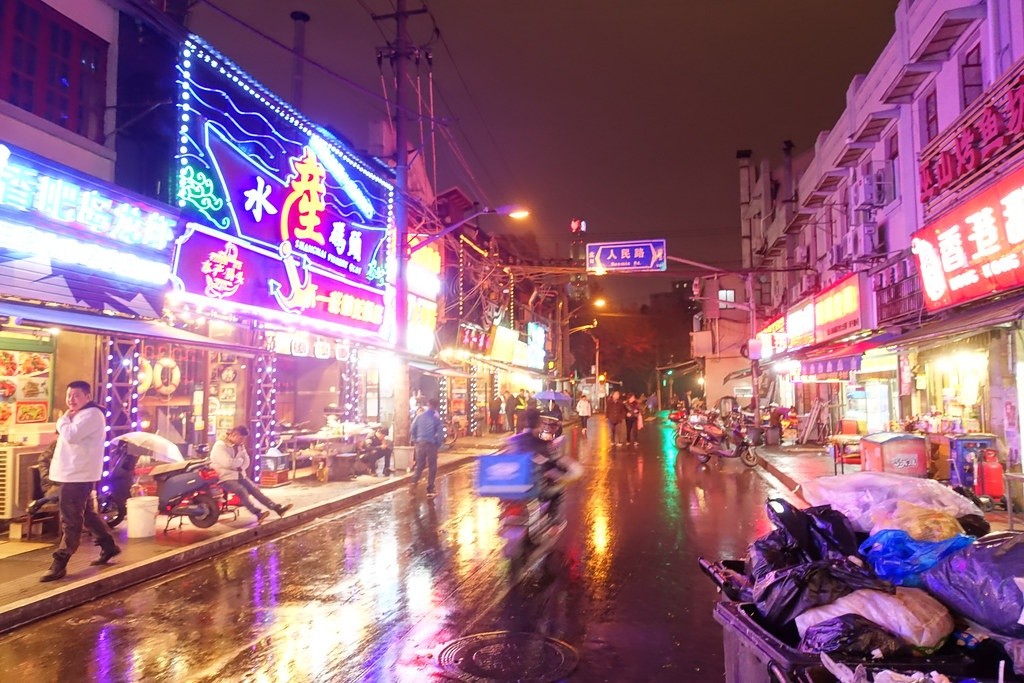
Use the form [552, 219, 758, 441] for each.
[392, 204, 532, 445]
[554, 295, 606, 393]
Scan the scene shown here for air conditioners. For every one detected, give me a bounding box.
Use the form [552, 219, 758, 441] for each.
[0, 446, 48, 519]
[792, 222, 878, 303]
[851, 175, 878, 211]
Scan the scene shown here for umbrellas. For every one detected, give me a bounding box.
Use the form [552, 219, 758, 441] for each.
[110, 428, 185, 484]
[532, 389, 572, 411]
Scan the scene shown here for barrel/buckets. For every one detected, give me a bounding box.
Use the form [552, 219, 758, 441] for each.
[126, 495, 159, 539]
[392, 446, 415, 470]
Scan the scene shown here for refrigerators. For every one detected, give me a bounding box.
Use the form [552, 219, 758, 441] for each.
[858, 433, 927, 476]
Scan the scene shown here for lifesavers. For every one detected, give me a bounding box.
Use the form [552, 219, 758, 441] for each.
[153, 357, 181, 395]
[137, 356, 153, 395]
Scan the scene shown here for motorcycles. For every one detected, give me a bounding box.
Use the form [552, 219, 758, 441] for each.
[673, 403, 763, 467]
[496, 412, 564, 572]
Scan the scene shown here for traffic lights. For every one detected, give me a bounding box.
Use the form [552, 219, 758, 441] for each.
[599, 370, 607, 385]
[662, 378, 668, 387]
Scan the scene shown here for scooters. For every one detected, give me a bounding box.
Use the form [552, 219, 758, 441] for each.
[95, 456, 220, 533]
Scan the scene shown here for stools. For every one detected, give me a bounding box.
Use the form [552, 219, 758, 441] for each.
[217, 485, 239, 522]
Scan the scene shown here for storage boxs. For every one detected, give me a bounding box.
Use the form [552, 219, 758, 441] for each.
[260, 468, 289, 485]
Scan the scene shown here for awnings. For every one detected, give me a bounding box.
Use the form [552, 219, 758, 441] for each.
[723, 360, 781, 386]
[800, 331, 901, 375]
[879, 295, 1024, 350]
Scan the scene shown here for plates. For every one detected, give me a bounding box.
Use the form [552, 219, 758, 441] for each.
[21, 359, 50, 377]
[17, 404, 46, 421]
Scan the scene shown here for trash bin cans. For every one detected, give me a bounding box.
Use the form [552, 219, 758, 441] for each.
[766, 427, 781, 445]
[746, 427, 765, 446]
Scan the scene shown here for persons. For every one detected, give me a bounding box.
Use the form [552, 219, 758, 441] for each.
[359, 426, 396, 477]
[540, 400, 562, 438]
[38, 380, 122, 583]
[605, 390, 658, 447]
[411, 398, 445, 497]
[770, 405, 797, 442]
[515, 389, 527, 434]
[684, 387, 692, 417]
[691, 394, 702, 409]
[671, 391, 678, 409]
[488, 395, 503, 432]
[562, 390, 571, 421]
[503, 390, 518, 434]
[411, 407, 428, 470]
[409, 388, 430, 421]
[496, 407, 577, 538]
[576, 395, 591, 431]
[210, 425, 294, 522]
[525, 390, 536, 406]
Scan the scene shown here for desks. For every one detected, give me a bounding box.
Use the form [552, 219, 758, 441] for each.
[923, 432, 951, 481]
[834, 442, 860, 475]
[292, 433, 369, 479]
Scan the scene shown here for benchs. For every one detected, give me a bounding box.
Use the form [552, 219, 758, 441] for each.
[26, 467, 98, 541]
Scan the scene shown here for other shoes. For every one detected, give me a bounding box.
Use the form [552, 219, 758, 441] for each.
[383, 467, 396, 474]
[411, 480, 420, 487]
[279, 504, 293, 517]
[427, 492, 437, 498]
[370, 469, 377, 477]
[256, 511, 270, 524]
[38, 566, 66, 581]
[90, 545, 122, 566]
